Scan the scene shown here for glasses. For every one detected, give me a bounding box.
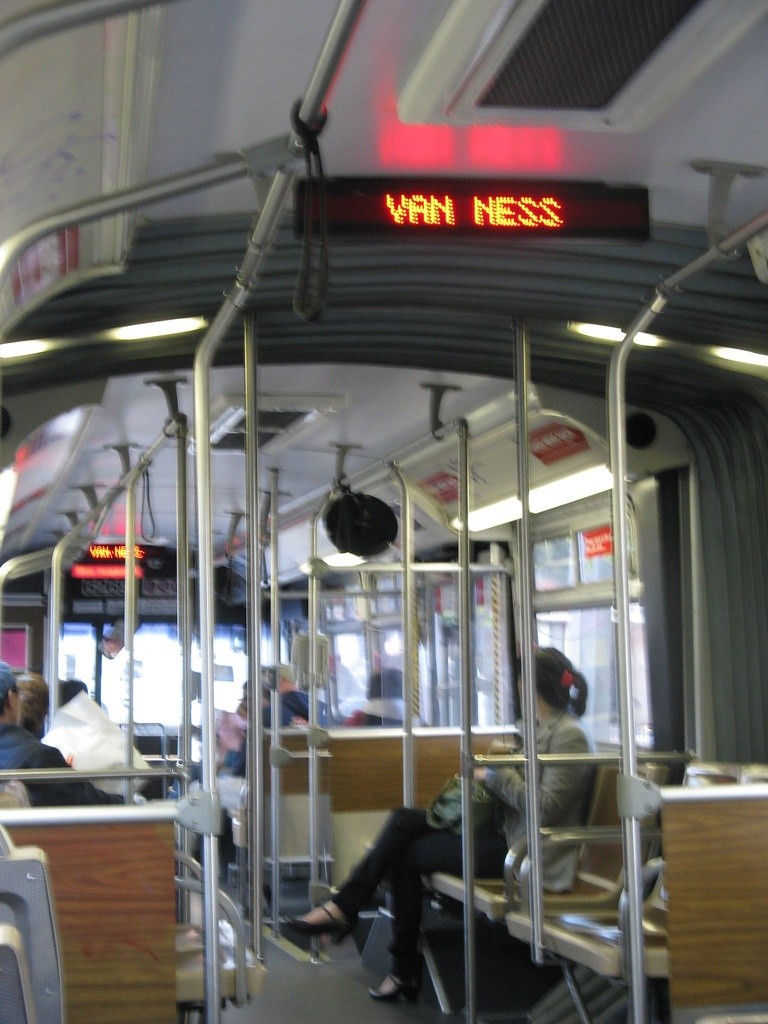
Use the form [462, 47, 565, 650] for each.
[102, 634, 112, 642]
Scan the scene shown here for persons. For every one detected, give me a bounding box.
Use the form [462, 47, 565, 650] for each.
[363, 652, 403, 726]
[189, 663, 329, 875]
[0, 661, 124, 808]
[101, 630, 123, 657]
[286, 646, 595, 1003]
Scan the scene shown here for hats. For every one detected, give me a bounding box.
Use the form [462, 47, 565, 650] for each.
[0, 658, 17, 695]
[16, 673, 50, 695]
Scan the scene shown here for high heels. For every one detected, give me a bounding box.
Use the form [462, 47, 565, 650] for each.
[286, 905, 357, 944]
[368, 973, 423, 1003]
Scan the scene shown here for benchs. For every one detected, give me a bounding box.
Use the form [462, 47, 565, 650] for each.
[0, 806, 268, 1024]
[229, 727, 514, 898]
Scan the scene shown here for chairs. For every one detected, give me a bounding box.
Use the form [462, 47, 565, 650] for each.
[419, 761, 768, 1023]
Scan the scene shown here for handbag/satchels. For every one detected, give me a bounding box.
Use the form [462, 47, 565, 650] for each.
[426, 774, 497, 845]
[39, 689, 152, 797]
